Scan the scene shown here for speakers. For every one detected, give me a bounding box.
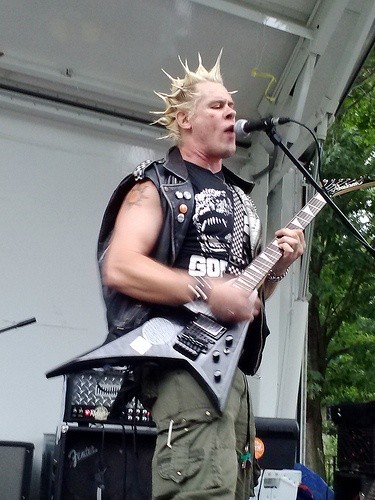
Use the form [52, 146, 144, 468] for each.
[55, 425, 158, 500]
[0, 441, 34, 500]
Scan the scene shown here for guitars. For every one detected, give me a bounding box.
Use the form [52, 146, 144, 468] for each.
[45, 175, 375, 417]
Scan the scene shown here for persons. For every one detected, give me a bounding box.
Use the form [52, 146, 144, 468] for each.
[97, 47, 307, 500]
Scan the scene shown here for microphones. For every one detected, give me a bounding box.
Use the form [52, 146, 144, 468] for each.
[234, 116, 290, 138]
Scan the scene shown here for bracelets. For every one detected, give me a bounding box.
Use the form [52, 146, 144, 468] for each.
[194, 277, 212, 306]
[267, 267, 290, 282]
